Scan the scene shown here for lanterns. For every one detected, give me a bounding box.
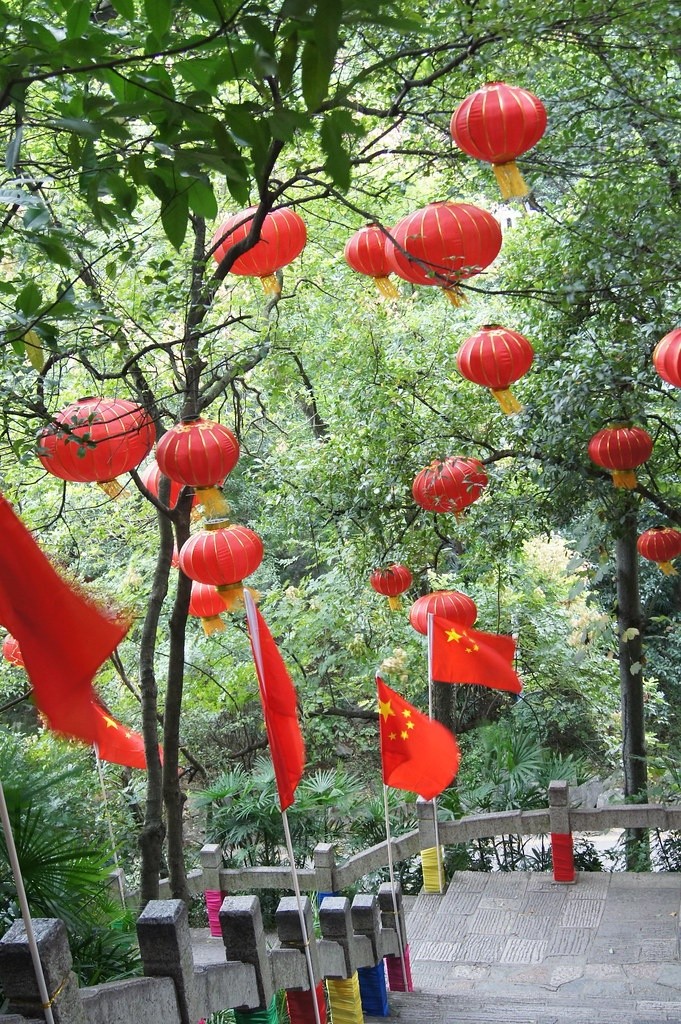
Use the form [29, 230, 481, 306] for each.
[212, 203, 307, 293]
[410, 590, 477, 636]
[39, 399, 156, 499]
[345, 223, 398, 300]
[170, 538, 180, 570]
[144, 459, 221, 520]
[458, 324, 533, 414]
[413, 456, 488, 524]
[3, 633, 25, 666]
[385, 201, 502, 308]
[157, 415, 240, 513]
[178, 518, 263, 610]
[654, 328, 681, 388]
[371, 562, 410, 611]
[450, 80, 547, 200]
[589, 421, 653, 489]
[188, 581, 227, 637]
[637, 525, 681, 576]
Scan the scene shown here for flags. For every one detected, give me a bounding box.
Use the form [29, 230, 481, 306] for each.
[0, 497, 129, 740]
[243, 590, 304, 812]
[426, 614, 522, 693]
[376, 675, 459, 801]
[90, 702, 166, 769]
